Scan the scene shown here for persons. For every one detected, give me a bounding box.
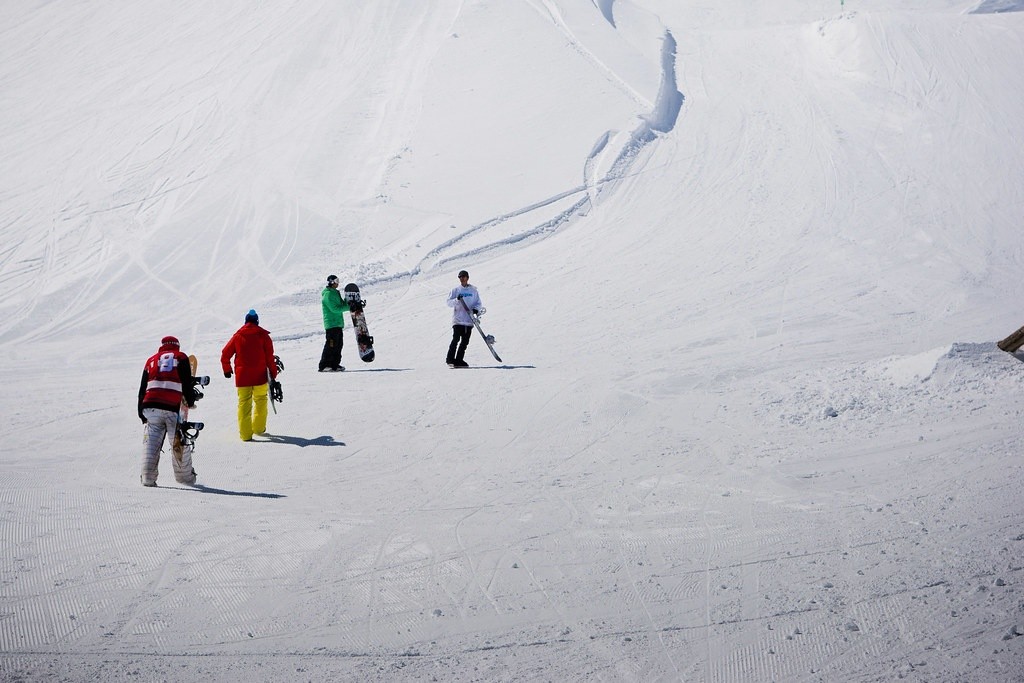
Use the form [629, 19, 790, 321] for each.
[446, 270, 481, 368]
[318, 275, 353, 373]
[220, 310, 277, 442]
[138, 336, 197, 487]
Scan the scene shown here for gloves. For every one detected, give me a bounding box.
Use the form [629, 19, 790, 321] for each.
[473, 309, 478, 314]
[457, 293, 463, 300]
[224, 371, 233, 378]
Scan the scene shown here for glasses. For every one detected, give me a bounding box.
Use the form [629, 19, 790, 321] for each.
[330, 277, 339, 284]
[458, 275, 468, 278]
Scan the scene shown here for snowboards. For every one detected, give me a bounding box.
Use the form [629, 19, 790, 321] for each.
[266, 355, 284, 414]
[344, 283, 375, 363]
[173, 355, 210, 468]
[460, 295, 503, 362]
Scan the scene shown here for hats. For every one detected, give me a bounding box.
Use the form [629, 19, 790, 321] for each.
[161, 336, 180, 350]
[327, 275, 339, 286]
[245, 309, 259, 326]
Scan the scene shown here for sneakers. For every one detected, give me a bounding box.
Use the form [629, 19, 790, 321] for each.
[319, 364, 345, 371]
[446, 356, 469, 368]
